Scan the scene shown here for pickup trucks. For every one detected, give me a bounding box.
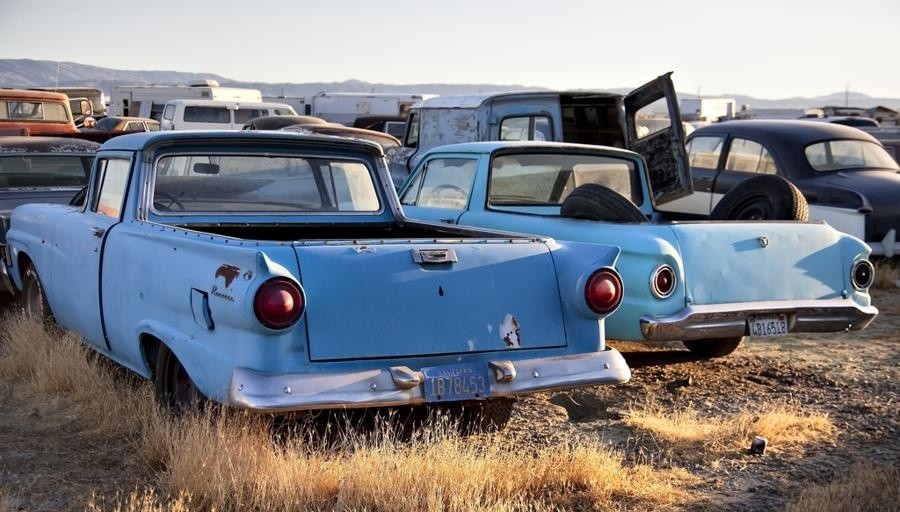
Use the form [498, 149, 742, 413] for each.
[2, 125, 637, 417]
[394, 139, 882, 360]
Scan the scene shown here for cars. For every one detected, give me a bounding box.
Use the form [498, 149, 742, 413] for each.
[657, 118, 900, 261]
[77, 116, 160, 133]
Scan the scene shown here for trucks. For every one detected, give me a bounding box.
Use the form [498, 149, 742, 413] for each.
[386, 67, 695, 207]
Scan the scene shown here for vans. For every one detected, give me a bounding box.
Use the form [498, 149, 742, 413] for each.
[158, 98, 299, 130]
